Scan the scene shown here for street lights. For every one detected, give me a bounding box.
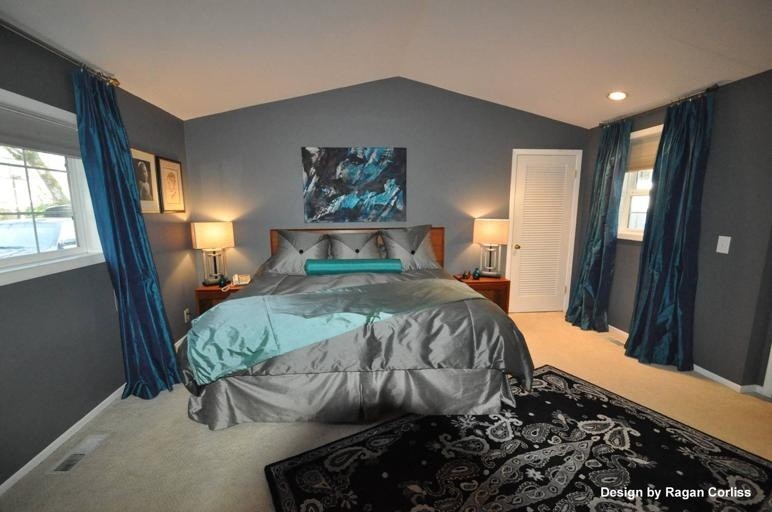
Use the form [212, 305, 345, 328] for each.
[11, 175, 21, 217]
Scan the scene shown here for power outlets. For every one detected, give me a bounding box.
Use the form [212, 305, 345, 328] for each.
[184, 308, 190, 323]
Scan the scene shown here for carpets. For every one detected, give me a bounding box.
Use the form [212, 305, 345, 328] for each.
[264, 363, 771, 512]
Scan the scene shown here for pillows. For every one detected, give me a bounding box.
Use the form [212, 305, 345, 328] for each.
[328, 231, 381, 259]
[271, 229, 328, 276]
[304, 258, 402, 276]
[378, 224, 442, 273]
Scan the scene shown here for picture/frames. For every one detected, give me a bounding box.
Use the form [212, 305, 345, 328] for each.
[131, 146, 160, 215]
[155, 155, 185, 215]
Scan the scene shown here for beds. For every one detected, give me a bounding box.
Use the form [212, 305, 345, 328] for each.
[174, 227, 535, 432]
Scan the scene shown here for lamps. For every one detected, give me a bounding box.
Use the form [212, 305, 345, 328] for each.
[473, 218, 510, 279]
[190, 221, 236, 286]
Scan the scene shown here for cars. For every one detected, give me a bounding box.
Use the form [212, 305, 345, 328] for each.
[37, 205, 78, 250]
[0, 219, 61, 260]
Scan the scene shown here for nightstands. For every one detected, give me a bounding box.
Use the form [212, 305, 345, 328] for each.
[195, 278, 252, 317]
[454, 273, 510, 316]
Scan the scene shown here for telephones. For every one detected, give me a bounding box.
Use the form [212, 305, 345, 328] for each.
[232, 274, 251, 286]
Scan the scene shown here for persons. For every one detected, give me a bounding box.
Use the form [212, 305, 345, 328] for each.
[138, 162, 151, 200]
